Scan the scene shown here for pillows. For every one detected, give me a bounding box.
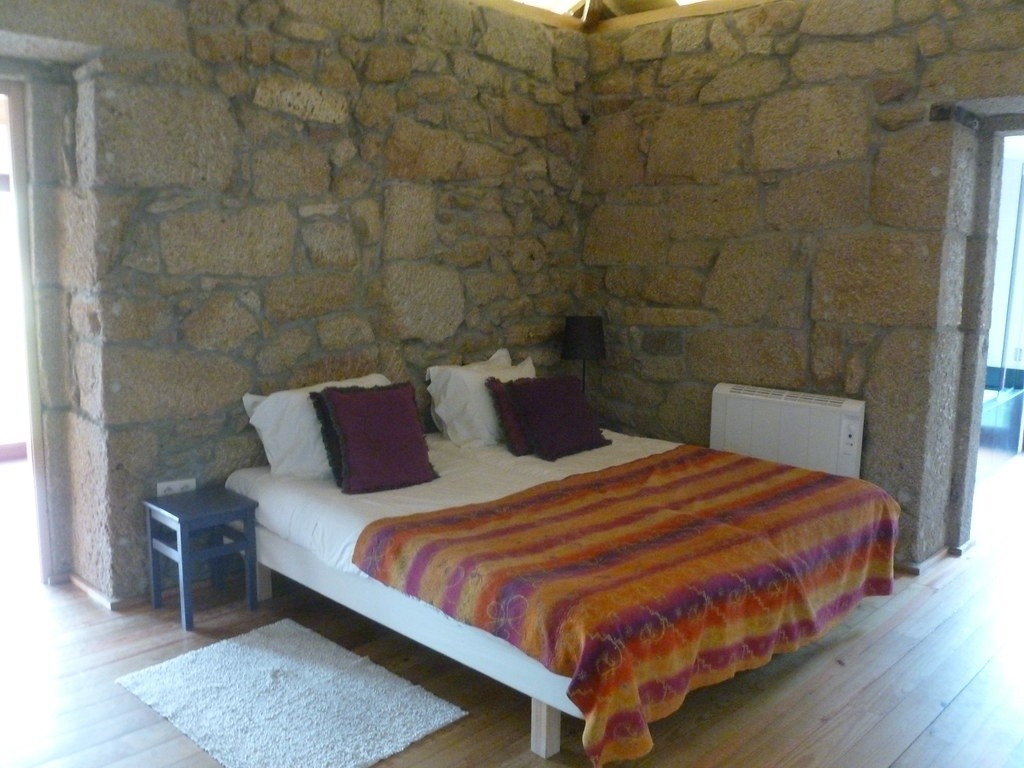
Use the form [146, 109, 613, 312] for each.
[243, 373, 392, 478]
[428, 343, 538, 448]
[484, 373, 612, 459]
[305, 380, 441, 495]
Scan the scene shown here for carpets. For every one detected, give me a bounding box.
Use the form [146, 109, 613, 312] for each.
[115, 619, 471, 768]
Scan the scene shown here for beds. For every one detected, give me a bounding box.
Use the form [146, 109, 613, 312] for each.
[222, 349, 903, 759]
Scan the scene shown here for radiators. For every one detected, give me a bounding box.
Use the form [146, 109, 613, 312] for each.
[707, 381, 867, 480]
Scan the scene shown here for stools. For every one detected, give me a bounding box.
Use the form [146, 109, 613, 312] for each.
[141, 487, 259, 633]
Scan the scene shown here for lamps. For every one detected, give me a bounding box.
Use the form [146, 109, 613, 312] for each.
[558, 314, 607, 398]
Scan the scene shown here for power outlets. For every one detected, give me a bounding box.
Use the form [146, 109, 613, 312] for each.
[153, 476, 198, 499]
